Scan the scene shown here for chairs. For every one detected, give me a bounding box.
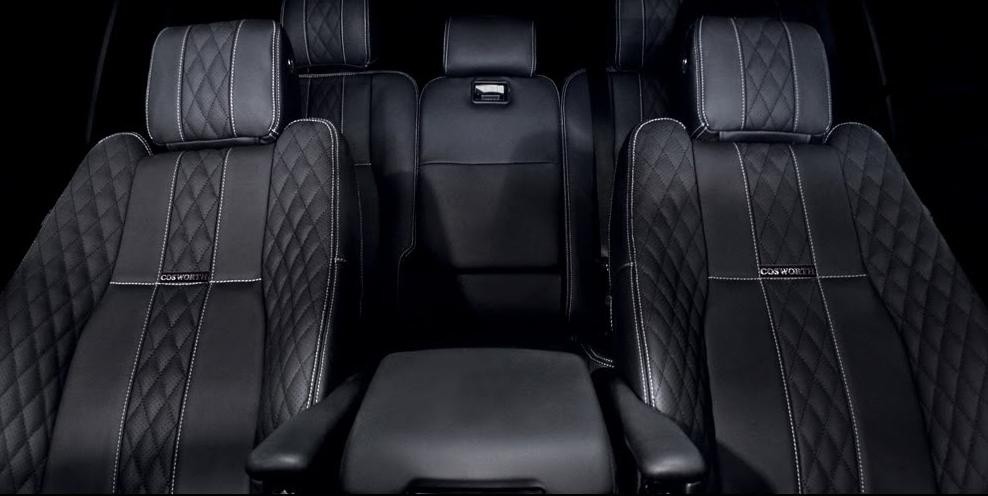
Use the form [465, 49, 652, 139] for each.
[272, 1, 694, 379]
[2, 13, 364, 496]
[609, 14, 987, 495]
